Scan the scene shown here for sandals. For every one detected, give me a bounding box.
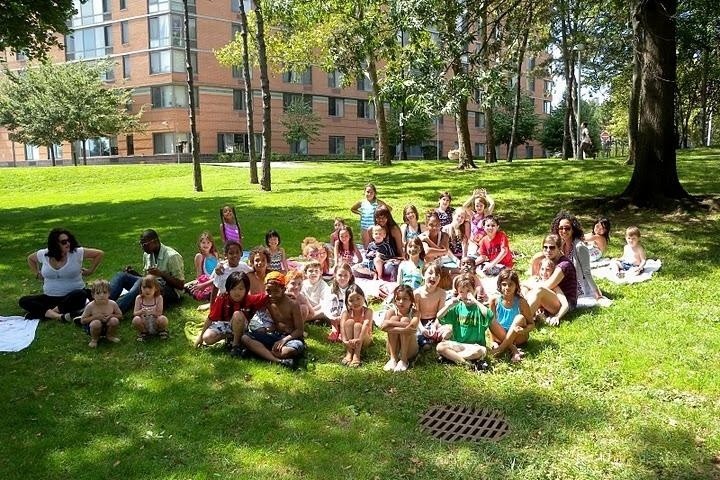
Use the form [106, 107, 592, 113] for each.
[341, 358, 361, 369]
[160, 329, 169, 341]
[137, 332, 148, 344]
[510, 348, 523, 364]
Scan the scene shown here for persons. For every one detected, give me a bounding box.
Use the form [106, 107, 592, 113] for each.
[20, 227, 104, 322]
[132, 275, 168, 341]
[81, 280, 123, 348]
[520, 213, 609, 324]
[610, 226, 646, 278]
[187, 181, 534, 369]
[577, 123, 596, 160]
[73, 228, 186, 323]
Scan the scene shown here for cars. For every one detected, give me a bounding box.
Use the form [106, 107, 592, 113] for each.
[101, 145, 120, 156]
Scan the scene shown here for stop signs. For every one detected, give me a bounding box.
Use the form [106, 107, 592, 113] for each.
[601, 131, 610, 142]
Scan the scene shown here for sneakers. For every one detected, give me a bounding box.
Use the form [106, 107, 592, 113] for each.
[226, 338, 251, 359]
[279, 358, 296, 371]
[24, 312, 39, 320]
[61, 312, 81, 323]
[436, 355, 455, 365]
[468, 360, 489, 373]
[382, 358, 409, 373]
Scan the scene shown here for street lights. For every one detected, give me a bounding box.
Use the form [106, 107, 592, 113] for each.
[436, 69, 443, 162]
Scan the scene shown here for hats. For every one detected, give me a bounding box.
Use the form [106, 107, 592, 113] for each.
[263, 271, 288, 287]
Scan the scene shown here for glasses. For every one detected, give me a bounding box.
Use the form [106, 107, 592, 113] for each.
[557, 228, 570, 231]
[58, 239, 70, 245]
[542, 246, 556, 251]
